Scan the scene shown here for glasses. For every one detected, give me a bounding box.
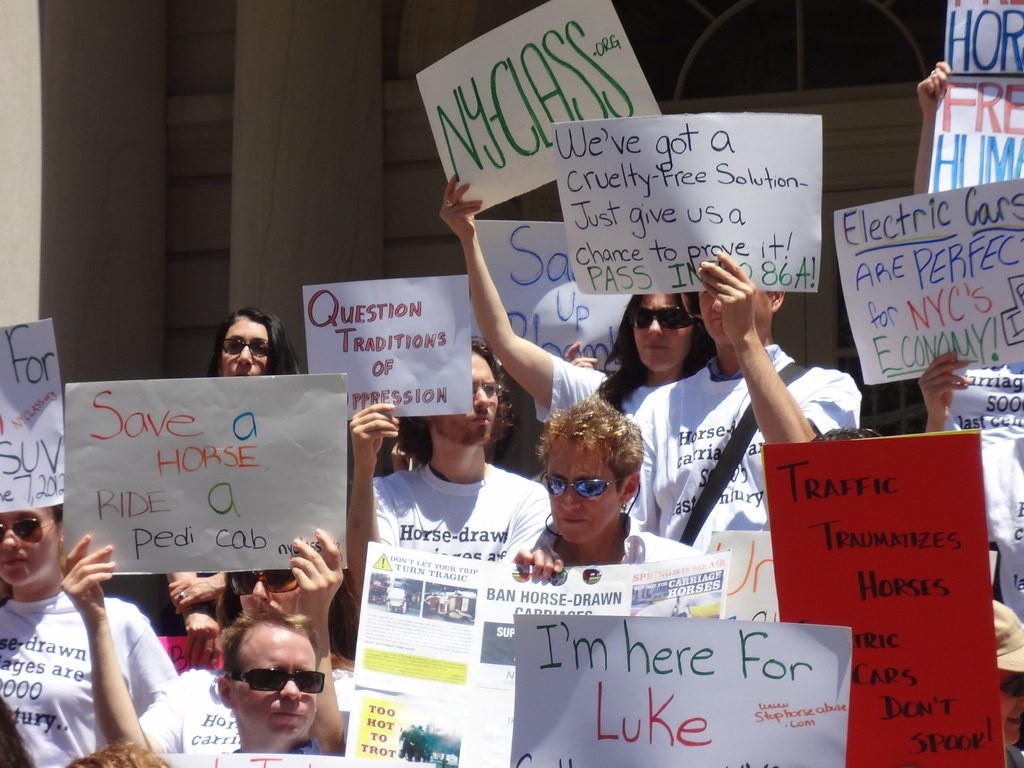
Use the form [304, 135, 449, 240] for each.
[629, 306, 695, 329]
[0, 518, 56, 544]
[225, 569, 299, 595]
[1000, 675, 1024, 697]
[222, 338, 271, 358]
[224, 669, 325, 693]
[473, 383, 505, 396]
[541, 470, 630, 499]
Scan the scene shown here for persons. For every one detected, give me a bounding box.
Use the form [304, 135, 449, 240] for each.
[0, 61, 1024, 768]
[0, 504, 179, 768]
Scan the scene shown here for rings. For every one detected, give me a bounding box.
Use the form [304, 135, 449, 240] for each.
[931, 70, 935, 74]
[179, 592, 185, 600]
[445, 200, 454, 207]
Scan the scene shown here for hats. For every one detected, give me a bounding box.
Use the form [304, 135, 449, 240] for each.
[992, 599, 1024, 673]
